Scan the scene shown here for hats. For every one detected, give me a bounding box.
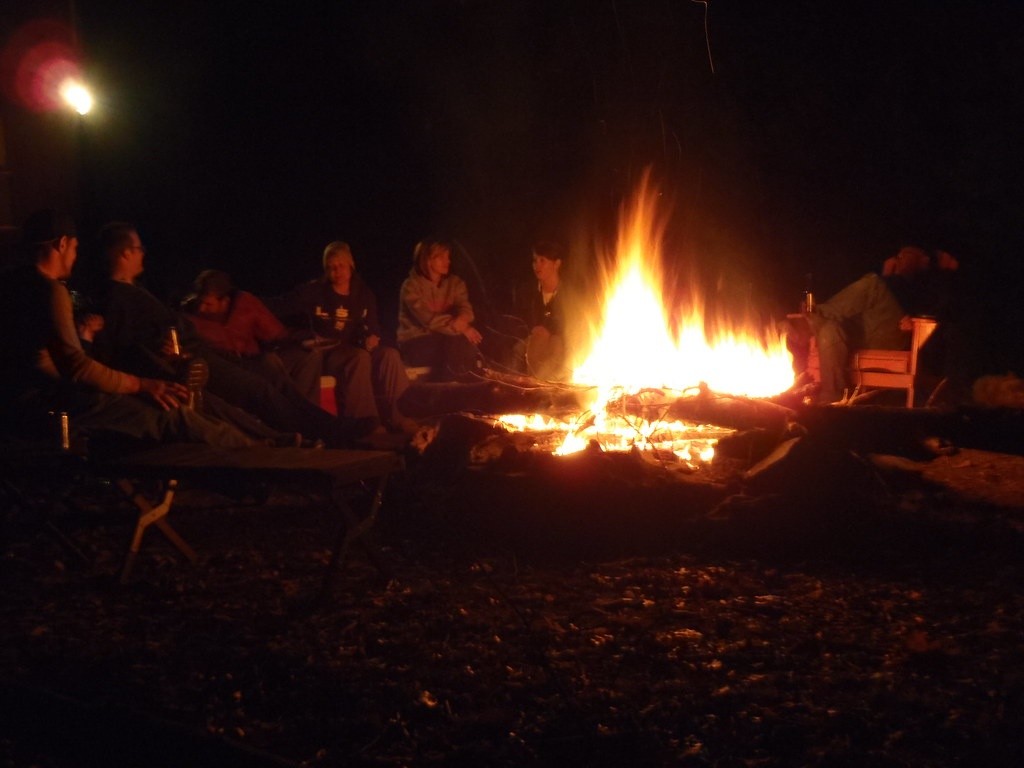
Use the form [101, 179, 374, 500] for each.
[12, 203, 79, 248]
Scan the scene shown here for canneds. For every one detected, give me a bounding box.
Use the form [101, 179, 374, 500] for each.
[48, 410, 69, 448]
[160, 326, 180, 356]
[801, 290, 812, 313]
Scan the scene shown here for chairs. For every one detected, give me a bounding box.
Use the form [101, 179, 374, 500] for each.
[0, 436, 395, 590]
[786, 309, 940, 410]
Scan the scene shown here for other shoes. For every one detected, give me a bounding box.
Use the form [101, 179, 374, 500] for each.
[361, 418, 418, 437]
[274, 432, 325, 449]
[803, 291, 821, 331]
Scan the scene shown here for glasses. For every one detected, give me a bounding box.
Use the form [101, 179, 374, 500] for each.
[133, 245, 144, 252]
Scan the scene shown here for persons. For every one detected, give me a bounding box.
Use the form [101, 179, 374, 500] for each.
[0, 209, 323, 451]
[279, 237, 422, 440]
[171, 264, 388, 443]
[68, 221, 325, 457]
[396, 235, 485, 367]
[505, 240, 583, 381]
[801, 243, 971, 405]
